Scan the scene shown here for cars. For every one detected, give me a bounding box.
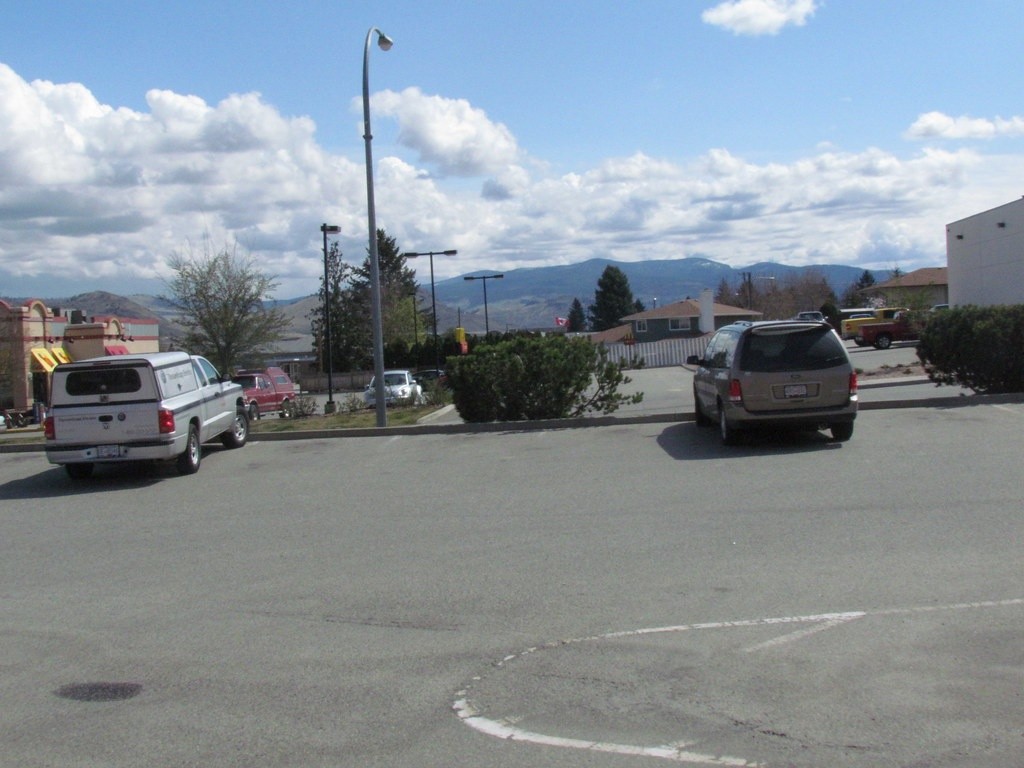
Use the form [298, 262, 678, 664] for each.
[364, 370, 422, 409]
[412, 370, 446, 391]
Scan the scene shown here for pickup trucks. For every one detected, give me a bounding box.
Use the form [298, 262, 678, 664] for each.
[44, 352, 250, 480]
[231, 366, 295, 421]
[841, 308, 909, 347]
[858, 310, 930, 349]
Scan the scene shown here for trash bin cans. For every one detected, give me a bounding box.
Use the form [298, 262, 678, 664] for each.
[33, 402, 45, 424]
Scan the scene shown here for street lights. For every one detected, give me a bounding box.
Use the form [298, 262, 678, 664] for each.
[362, 27, 394, 427]
[749, 272, 775, 308]
[464, 274, 504, 344]
[320, 223, 342, 414]
[653, 297, 656, 309]
[408, 292, 418, 347]
[404, 250, 457, 377]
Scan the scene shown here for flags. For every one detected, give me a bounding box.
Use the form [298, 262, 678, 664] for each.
[555, 316, 570, 327]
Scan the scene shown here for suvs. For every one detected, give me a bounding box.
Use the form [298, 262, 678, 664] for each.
[929, 304, 949, 320]
[797, 311, 828, 323]
[687, 321, 859, 447]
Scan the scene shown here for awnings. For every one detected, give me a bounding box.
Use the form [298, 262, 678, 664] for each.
[30, 348, 59, 408]
[52, 347, 72, 364]
[105, 345, 129, 356]
